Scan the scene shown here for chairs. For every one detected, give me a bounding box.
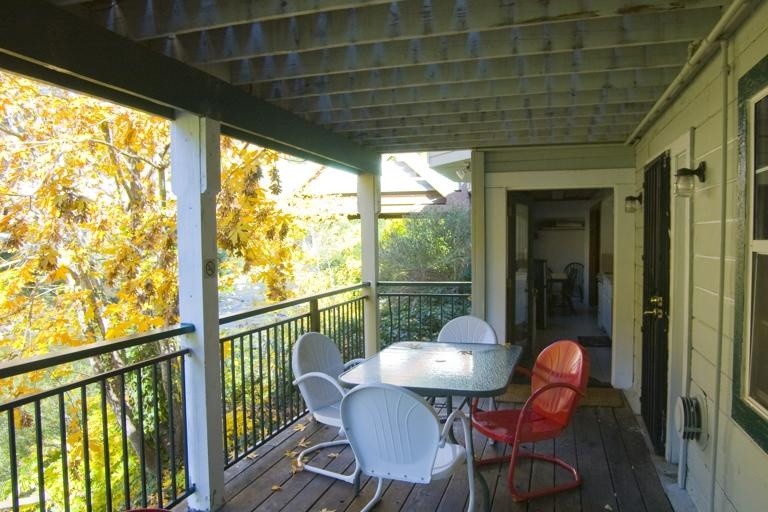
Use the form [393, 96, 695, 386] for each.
[551, 262, 584, 316]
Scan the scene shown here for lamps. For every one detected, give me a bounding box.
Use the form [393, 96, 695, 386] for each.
[674, 161, 707, 198]
[623, 192, 642, 214]
